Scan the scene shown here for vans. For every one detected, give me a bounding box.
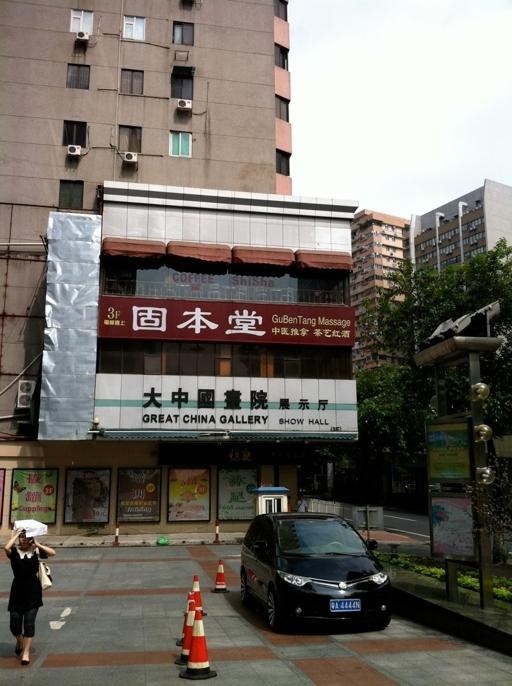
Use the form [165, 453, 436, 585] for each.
[238, 509, 395, 634]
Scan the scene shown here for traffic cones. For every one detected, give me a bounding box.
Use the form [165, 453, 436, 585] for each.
[171, 590, 196, 648]
[173, 598, 197, 668]
[210, 558, 230, 593]
[176, 604, 218, 683]
[182, 573, 209, 616]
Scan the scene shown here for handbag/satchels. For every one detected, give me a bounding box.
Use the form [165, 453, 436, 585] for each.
[35, 547, 52, 590]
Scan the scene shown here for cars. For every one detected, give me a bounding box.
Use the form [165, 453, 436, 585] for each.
[439, 481, 474, 494]
[429, 482, 442, 493]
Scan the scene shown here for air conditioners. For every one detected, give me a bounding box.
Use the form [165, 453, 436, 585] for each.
[68, 144, 82, 156]
[16, 379, 37, 409]
[123, 151, 139, 163]
[177, 97, 193, 111]
[76, 30, 90, 42]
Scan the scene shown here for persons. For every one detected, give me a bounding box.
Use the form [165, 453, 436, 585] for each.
[4, 530, 56, 665]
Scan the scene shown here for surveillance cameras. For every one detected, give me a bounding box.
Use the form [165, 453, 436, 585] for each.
[94, 417, 99, 425]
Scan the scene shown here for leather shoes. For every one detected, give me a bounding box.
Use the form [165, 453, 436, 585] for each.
[16, 648, 30, 665]
